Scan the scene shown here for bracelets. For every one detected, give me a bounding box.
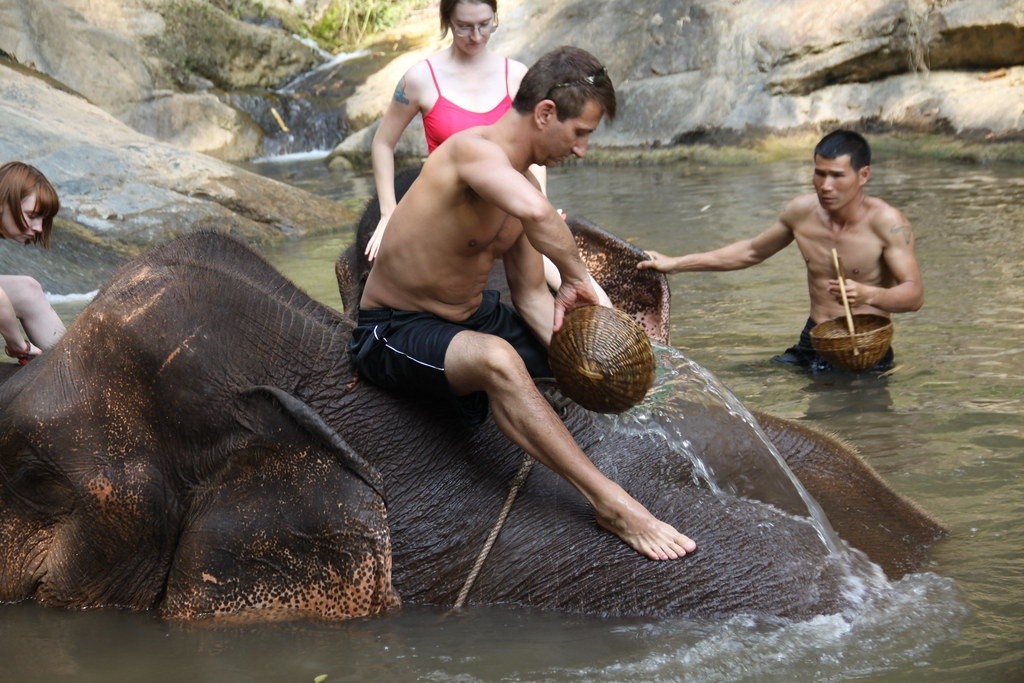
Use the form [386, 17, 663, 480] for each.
[5, 339, 31, 358]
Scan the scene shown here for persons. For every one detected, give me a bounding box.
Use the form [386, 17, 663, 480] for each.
[363, 0, 611, 305]
[351, 44, 700, 564]
[0, 161, 70, 369]
[636, 126, 922, 372]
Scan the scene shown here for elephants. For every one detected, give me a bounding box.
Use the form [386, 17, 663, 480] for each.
[0, 171, 948, 624]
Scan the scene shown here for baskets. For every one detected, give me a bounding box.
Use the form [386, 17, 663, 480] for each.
[810, 248, 893, 374]
[548, 305, 656, 415]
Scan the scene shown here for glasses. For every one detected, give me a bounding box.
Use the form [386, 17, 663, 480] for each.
[554, 66, 608, 89]
[449, 12, 498, 37]
